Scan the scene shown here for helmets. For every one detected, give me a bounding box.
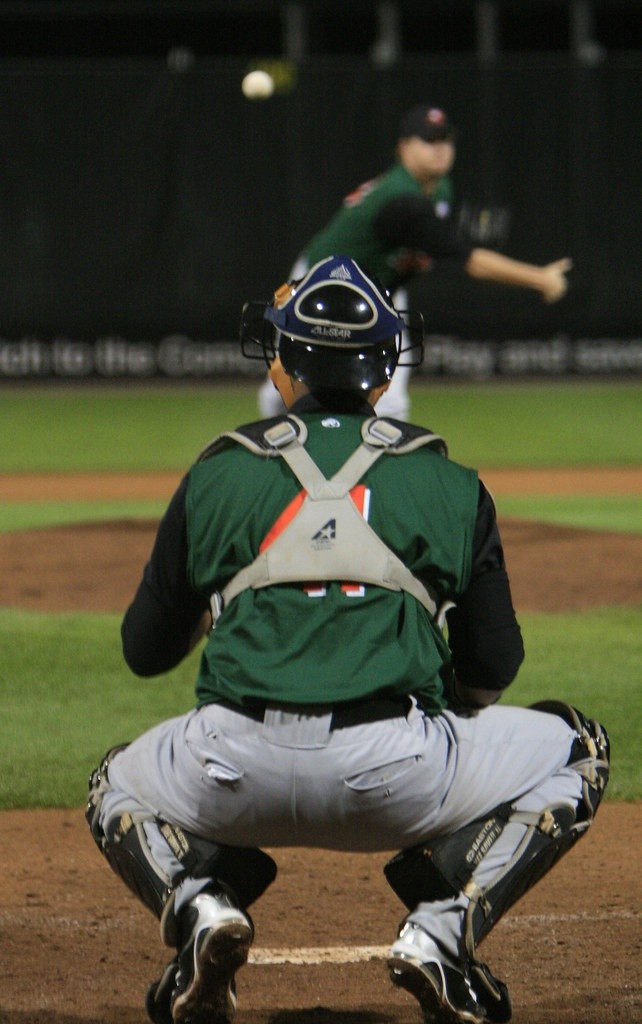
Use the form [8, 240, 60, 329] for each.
[274, 274, 398, 389]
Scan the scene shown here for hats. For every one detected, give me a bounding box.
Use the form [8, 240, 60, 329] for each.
[403, 103, 456, 140]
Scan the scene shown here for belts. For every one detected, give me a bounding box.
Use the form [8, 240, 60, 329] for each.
[216, 693, 430, 728]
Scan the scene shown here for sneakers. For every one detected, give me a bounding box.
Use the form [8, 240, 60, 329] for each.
[385, 921, 490, 1024]
[157, 891, 255, 1024]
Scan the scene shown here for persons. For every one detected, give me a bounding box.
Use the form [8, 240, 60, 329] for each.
[86, 258, 608, 1024]
[256, 104, 567, 423]
